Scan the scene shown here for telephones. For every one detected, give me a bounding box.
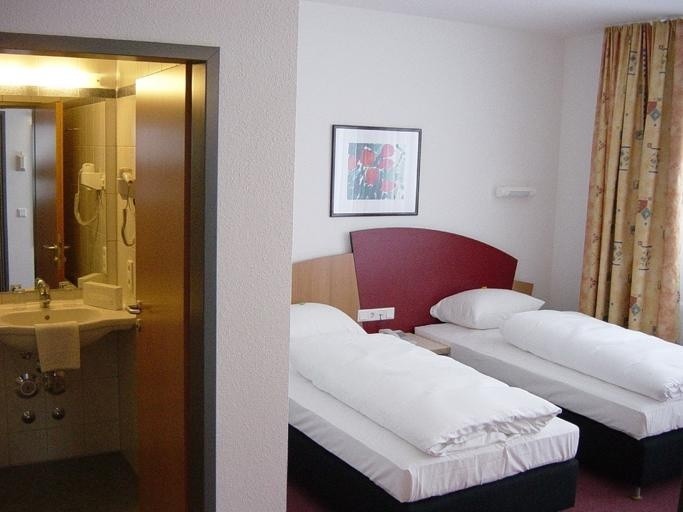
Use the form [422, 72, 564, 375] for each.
[379, 328, 406, 339]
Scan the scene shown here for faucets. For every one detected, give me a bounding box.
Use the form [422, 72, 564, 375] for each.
[79, 163, 95, 192]
[115, 167, 133, 201]
[37, 282, 52, 308]
[34, 277, 45, 287]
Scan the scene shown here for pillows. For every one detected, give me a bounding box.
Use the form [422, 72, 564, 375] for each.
[428, 287, 547, 330]
[287, 299, 368, 364]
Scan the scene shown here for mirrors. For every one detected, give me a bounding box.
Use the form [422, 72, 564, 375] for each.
[0, 95, 108, 295]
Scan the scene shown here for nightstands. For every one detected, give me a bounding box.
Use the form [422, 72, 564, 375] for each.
[394, 331, 452, 356]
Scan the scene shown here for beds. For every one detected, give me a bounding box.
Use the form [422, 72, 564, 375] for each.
[284, 335, 583, 512]
[413, 308, 681, 501]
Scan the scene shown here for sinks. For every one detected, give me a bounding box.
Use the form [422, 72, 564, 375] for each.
[0, 299, 138, 354]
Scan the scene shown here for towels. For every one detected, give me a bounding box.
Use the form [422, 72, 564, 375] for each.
[34, 320, 82, 373]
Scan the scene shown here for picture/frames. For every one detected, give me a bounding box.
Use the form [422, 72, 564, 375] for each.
[328, 123, 422, 218]
